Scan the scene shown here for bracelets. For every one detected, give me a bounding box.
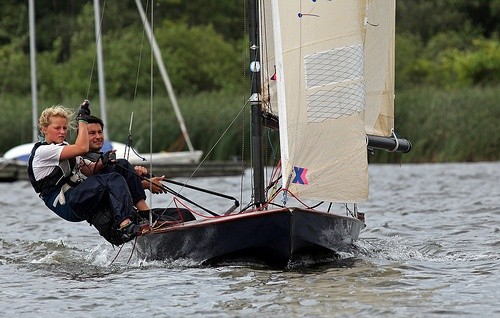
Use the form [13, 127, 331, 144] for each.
[78, 120, 88, 124]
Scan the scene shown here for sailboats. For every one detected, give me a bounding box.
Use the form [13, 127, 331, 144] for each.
[2, 1, 204, 166]
[74, 0, 412, 272]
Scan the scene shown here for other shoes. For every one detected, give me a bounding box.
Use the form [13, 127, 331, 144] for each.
[139, 212, 176, 223]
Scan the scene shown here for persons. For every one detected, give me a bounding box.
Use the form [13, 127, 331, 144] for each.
[28, 100, 149, 246]
[72, 115, 179, 226]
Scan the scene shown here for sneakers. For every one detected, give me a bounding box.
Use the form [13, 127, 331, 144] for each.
[117, 221, 150, 243]
